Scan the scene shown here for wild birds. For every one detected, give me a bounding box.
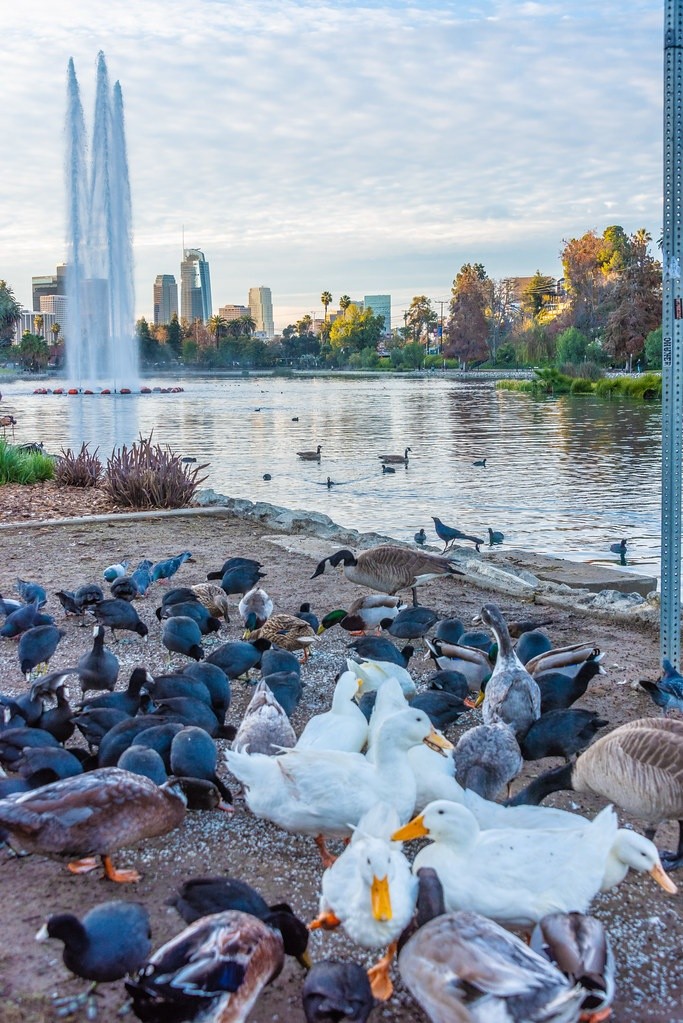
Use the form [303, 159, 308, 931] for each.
[181, 457, 196, 463]
[122, 912, 311, 1023]
[472, 458, 487, 467]
[529, 909, 616, 1022]
[34, 898, 154, 1018]
[263, 474, 271, 479]
[377, 447, 413, 463]
[487, 526, 504, 545]
[430, 517, 484, 555]
[326, 477, 334, 484]
[381, 465, 395, 473]
[610, 538, 629, 554]
[398, 869, 585, 1023]
[415, 528, 426, 545]
[162, 876, 296, 926]
[296, 445, 323, 458]
[0, 546, 683, 788]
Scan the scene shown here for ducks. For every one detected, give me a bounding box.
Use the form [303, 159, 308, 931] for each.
[269, 671, 369, 755]
[0, 766, 234, 882]
[465, 785, 678, 896]
[499, 718, 683, 874]
[390, 799, 618, 946]
[220, 709, 457, 865]
[306, 801, 420, 1001]
